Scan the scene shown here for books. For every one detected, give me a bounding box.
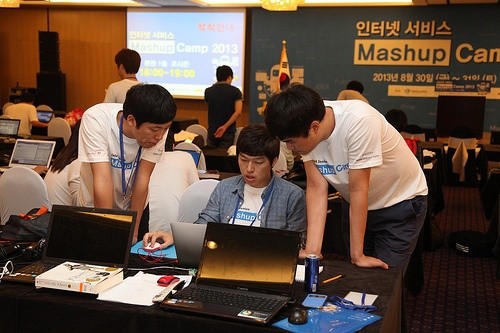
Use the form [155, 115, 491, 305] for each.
[35, 261, 123, 294]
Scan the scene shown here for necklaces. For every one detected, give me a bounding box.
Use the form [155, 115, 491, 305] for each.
[122, 77, 136, 80]
[119, 114, 142, 211]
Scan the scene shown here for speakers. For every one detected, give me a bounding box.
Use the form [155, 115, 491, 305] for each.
[39, 31, 59, 71]
[37, 71, 67, 119]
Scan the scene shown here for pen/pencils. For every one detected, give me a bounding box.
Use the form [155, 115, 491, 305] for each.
[322, 274, 345, 283]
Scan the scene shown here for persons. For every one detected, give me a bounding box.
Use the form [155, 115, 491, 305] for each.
[143, 122, 307, 250]
[104, 49, 141, 103]
[77, 84, 177, 245]
[337, 81, 369, 104]
[205, 65, 242, 148]
[4, 92, 49, 139]
[385, 108, 415, 139]
[264, 84, 428, 268]
[0, 164, 48, 177]
[43, 118, 81, 207]
[149, 128, 199, 234]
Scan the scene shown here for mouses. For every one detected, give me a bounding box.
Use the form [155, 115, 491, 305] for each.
[288, 303, 307, 324]
[143, 242, 161, 252]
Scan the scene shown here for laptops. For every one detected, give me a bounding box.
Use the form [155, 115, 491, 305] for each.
[37, 110, 53, 122]
[161, 221, 302, 327]
[1, 204, 136, 283]
[8, 139, 56, 169]
[0, 119, 20, 137]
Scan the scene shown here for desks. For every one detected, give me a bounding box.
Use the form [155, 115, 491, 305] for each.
[0, 118, 500, 333]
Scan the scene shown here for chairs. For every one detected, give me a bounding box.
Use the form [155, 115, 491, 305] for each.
[186, 124, 207, 144]
[178, 178, 219, 223]
[175, 142, 207, 171]
[47, 118, 71, 146]
[0, 166, 49, 225]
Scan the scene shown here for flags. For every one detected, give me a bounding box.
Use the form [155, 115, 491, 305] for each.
[278, 45, 291, 90]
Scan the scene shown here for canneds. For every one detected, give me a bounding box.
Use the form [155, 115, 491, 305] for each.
[305, 254, 320, 294]
